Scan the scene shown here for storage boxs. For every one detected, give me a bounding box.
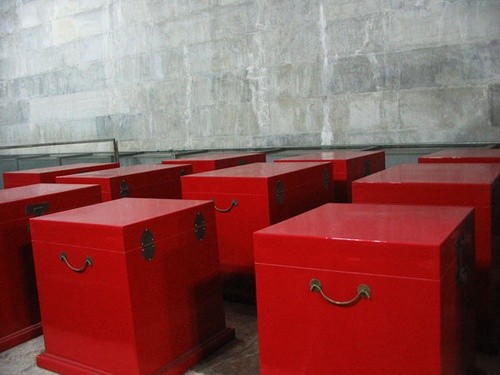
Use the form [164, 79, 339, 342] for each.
[0, 145, 500, 375]
